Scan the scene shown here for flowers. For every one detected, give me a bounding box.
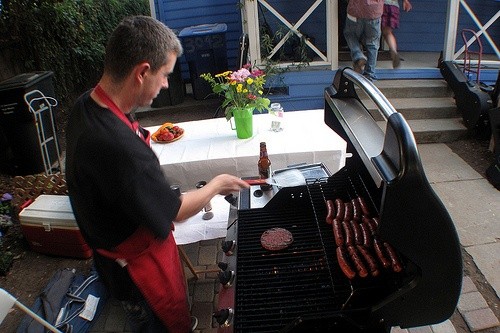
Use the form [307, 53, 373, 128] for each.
[0, 190, 16, 278]
[200, 63, 271, 122]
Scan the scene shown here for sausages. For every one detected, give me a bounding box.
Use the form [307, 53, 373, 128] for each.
[336, 239, 402, 279]
[332, 216, 380, 248]
[325, 197, 370, 224]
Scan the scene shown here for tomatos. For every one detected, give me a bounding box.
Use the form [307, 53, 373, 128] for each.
[157, 126, 180, 141]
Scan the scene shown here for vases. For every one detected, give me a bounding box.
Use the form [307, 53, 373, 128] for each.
[232, 109, 252, 138]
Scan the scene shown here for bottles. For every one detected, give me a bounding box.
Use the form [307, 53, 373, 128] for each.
[269, 103, 284, 132]
[258, 142, 272, 191]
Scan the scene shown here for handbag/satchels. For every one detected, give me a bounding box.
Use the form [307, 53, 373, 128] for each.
[48, 267, 110, 333]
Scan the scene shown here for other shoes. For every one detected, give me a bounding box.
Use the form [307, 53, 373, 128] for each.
[392, 51, 400, 68]
[354, 58, 367, 75]
[192, 316, 198, 331]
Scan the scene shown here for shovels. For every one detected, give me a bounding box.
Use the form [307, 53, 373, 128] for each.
[245, 168, 306, 187]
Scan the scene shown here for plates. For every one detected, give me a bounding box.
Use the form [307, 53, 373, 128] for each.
[151, 129, 184, 144]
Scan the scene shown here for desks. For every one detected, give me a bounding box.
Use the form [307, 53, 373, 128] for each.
[143, 109, 348, 193]
[169, 194, 235, 280]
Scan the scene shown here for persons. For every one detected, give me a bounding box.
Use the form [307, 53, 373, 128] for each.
[65, 15, 251, 333]
[344, 0, 384, 86]
[381, 0, 412, 68]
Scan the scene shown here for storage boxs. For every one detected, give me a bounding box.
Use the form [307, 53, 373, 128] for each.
[18, 194, 94, 259]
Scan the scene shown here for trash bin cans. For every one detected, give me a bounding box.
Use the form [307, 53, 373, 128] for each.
[178, 23, 229, 99]
[151, 29, 186, 108]
[0, 71, 62, 176]
